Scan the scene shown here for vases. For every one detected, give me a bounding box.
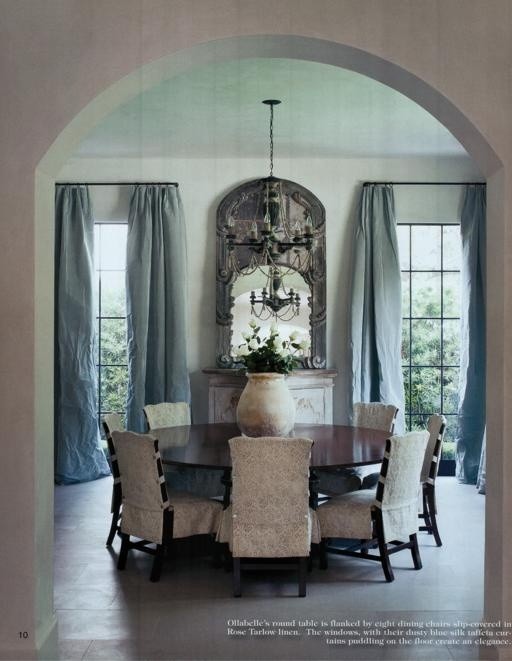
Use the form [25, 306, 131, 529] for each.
[235, 372, 295, 438]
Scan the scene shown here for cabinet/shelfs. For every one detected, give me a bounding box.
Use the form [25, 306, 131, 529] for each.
[202, 364, 339, 425]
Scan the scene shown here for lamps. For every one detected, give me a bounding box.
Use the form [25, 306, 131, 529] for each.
[217, 96, 325, 321]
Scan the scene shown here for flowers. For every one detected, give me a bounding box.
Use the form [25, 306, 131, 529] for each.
[228, 320, 309, 374]
